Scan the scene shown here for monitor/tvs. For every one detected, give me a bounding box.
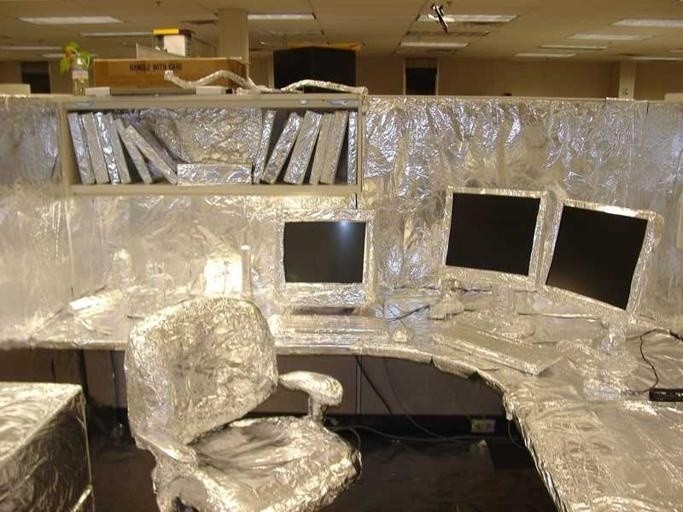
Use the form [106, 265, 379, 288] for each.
[539, 196, 664, 320]
[274, 207, 374, 306]
[440, 184, 549, 293]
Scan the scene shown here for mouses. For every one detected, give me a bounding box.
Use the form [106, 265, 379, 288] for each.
[391, 326, 416, 343]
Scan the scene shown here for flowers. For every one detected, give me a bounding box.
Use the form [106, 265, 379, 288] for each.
[56, 39, 90, 69]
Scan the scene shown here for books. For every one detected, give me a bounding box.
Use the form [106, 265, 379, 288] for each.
[261, 111, 302, 184]
[281, 110, 323, 185]
[335, 111, 357, 184]
[66, 111, 177, 186]
[308, 112, 334, 185]
[319, 108, 349, 184]
[251, 109, 277, 185]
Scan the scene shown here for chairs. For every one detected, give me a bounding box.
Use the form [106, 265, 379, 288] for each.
[120, 294, 362, 512]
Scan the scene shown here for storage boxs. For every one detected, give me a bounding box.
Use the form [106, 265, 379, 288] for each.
[90, 56, 248, 94]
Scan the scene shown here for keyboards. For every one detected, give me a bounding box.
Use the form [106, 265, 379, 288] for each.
[431, 321, 564, 377]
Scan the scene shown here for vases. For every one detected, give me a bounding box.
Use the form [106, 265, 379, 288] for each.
[68, 63, 87, 97]
